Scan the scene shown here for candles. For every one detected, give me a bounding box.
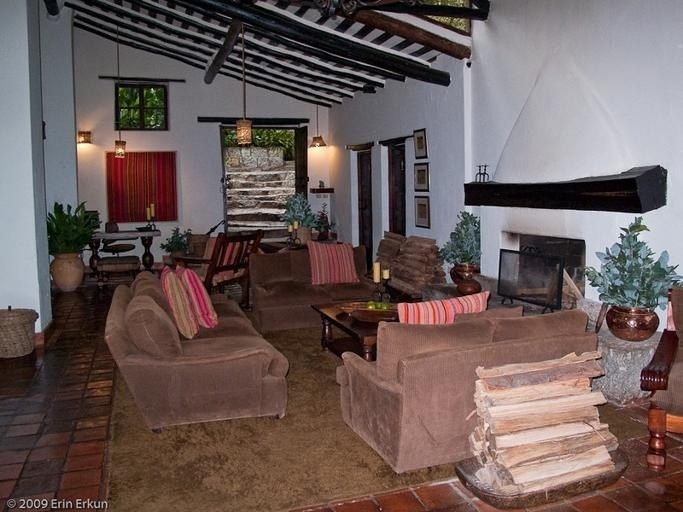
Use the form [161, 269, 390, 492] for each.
[146, 207, 151, 221]
[382, 269, 389, 280]
[373, 261, 381, 284]
[288, 223, 293, 233]
[293, 220, 298, 230]
[150, 203, 155, 218]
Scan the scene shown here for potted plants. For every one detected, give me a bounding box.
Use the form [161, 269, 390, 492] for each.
[47, 201, 102, 292]
[584, 217, 683, 341]
[160, 227, 192, 268]
[437, 211, 482, 285]
[278, 191, 336, 245]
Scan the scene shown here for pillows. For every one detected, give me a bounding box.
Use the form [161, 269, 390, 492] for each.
[176, 265, 218, 329]
[306, 240, 360, 286]
[454, 305, 525, 322]
[161, 265, 199, 339]
[398, 291, 491, 325]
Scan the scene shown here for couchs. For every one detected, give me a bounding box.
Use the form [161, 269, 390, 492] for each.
[103, 271, 290, 434]
[336, 310, 600, 473]
[248, 246, 380, 337]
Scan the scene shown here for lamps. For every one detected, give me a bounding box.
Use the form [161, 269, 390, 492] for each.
[310, 103, 327, 147]
[236, 22, 252, 144]
[114, 22, 125, 160]
[78, 131, 92, 144]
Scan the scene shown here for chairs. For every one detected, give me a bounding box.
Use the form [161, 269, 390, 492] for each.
[102, 221, 135, 256]
[175, 229, 266, 308]
[641, 287, 683, 471]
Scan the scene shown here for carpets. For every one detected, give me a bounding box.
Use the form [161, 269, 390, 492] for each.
[102, 309, 649, 512]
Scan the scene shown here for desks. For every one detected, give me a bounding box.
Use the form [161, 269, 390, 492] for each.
[88, 230, 160, 290]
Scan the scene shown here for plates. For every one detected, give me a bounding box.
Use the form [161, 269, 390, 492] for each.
[136, 227, 152, 231]
[335, 302, 398, 322]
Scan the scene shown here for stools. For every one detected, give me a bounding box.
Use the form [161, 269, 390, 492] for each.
[97, 256, 140, 287]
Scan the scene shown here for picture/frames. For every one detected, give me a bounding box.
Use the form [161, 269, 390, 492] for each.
[413, 128, 431, 159]
[414, 196, 431, 229]
[413, 162, 429, 192]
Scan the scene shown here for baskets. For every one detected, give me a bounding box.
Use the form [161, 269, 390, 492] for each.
[0, 309, 38, 358]
[187, 234, 210, 257]
